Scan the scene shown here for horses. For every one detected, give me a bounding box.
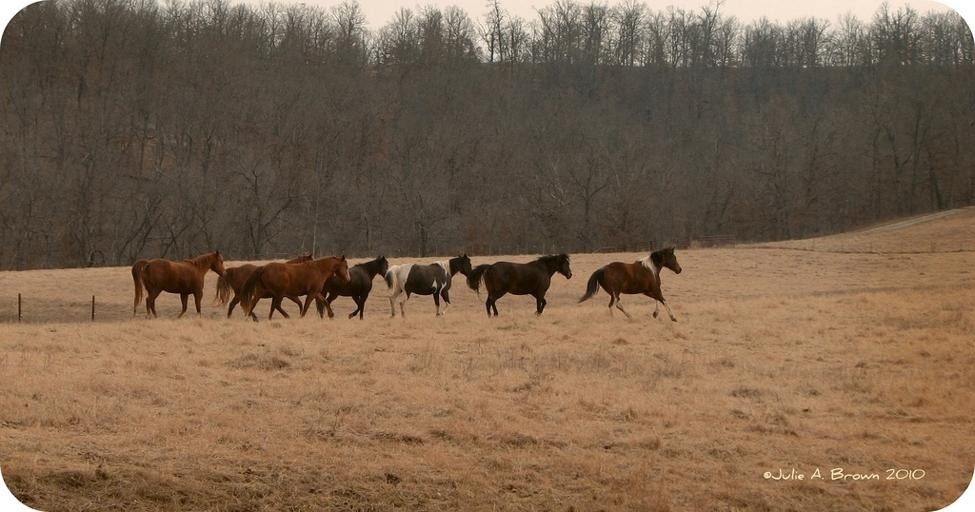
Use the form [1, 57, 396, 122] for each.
[465, 252, 573, 318]
[576, 246, 682, 322]
[131, 248, 228, 321]
[211, 254, 389, 320]
[383, 253, 473, 322]
